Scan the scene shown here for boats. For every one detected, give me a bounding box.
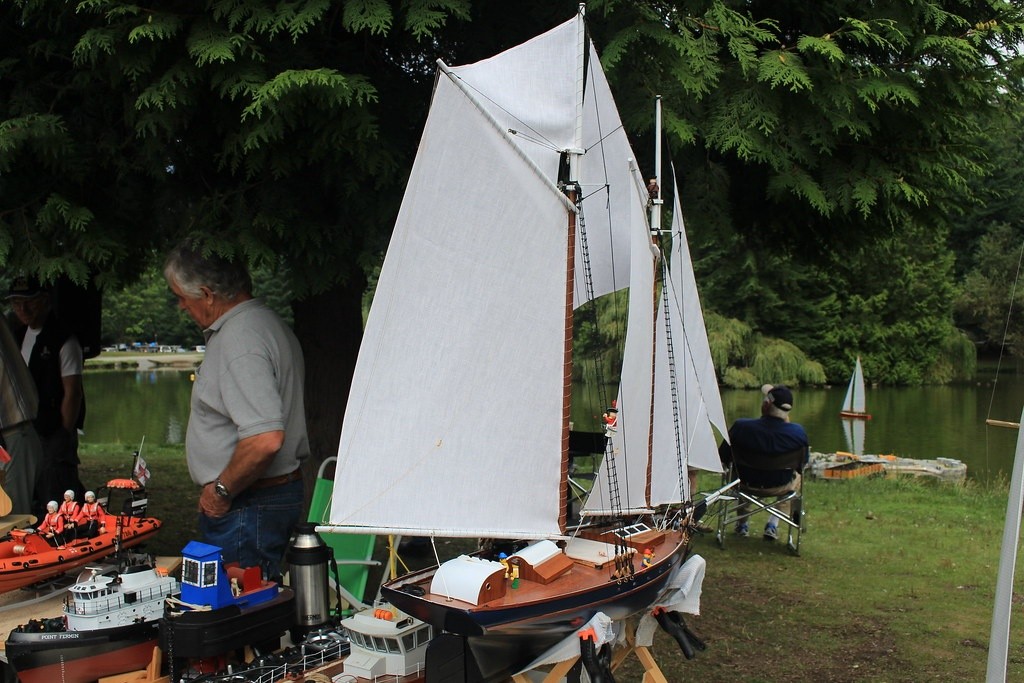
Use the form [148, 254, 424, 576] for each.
[0, 434, 163, 595]
[340, 603, 431, 680]
[5, 512, 294, 683]
[808, 451, 968, 486]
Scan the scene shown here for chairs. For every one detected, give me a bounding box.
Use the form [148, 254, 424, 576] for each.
[306, 456, 381, 616]
[715, 442, 808, 556]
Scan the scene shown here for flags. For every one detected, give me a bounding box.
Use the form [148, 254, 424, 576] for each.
[134, 457, 150, 485]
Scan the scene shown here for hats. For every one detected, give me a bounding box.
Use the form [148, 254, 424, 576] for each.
[5, 276, 40, 300]
[761, 382, 793, 412]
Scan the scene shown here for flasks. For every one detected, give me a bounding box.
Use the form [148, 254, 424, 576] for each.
[287, 522, 340, 644]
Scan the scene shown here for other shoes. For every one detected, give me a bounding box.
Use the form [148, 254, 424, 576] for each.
[697, 522, 714, 532]
[763, 524, 778, 541]
[735, 521, 751, 538]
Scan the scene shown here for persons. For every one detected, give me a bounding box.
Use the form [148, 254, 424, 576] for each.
[498, 552, 510, 582]
[641, 548, 652, 569]
[58, 489, 80, 541]
[602, 408, 619, 437]
[38, 500, 64, 547]
[0, 274, 86, 590]
[509, 558, 520, 589]
[68, 490, 106, 539]
[163, 234, 311, 660]
[718, 384, 809, 542]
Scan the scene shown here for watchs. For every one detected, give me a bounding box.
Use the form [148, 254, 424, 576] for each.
[215, 477, 232, 499]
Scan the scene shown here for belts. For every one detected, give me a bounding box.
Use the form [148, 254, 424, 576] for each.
[252, 467, 303, 488]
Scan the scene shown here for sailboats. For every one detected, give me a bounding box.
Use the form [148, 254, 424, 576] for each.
[840, 356, 871, 417]
[312, 3, 732, 655]
